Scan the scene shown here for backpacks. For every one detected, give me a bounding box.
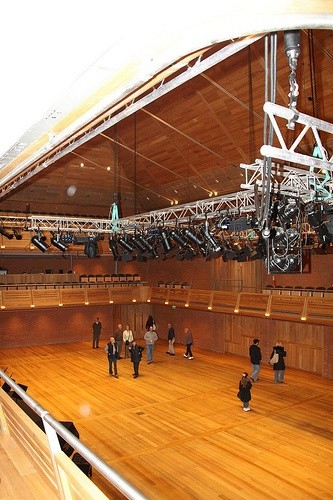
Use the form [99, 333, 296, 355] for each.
[139, 349, 142, 359]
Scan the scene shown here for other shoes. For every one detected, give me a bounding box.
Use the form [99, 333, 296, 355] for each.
[275, 379, 283, 382]
[165, 352, 175, 355]
[111, 375, 119, 379]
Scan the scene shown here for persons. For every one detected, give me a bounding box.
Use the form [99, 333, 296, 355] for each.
[166, 323, 176, 355]
[249, 339, 262, 381]
[114, 324, 123, 359]
[237, 372, 252, 412]
[144, 326, 158, 364]
[183, 327, 194, 360]
[92, 316, 102, 349]
[268, 341, 286, 384]
[122, 325, 133, 359]
[146, 315, 156, 332]
[128, 340, 144, 378]
[105, 337, 119, 378]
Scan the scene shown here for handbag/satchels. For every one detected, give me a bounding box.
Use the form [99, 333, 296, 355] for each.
[152, 319, 157, 331]
[269, 349, 278, 365]
[126, 340, 129, 345]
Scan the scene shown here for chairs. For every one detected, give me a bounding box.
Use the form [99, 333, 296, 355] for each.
[0, 274, 143, 287]
[158, 281, 191, 289]
[262, 285, 333, 298]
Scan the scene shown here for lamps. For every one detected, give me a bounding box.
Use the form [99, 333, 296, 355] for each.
[284, 29, 301, 131]
[0, 198, 333, 271]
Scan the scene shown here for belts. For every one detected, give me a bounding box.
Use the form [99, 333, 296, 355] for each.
[116, 339, 123, 341]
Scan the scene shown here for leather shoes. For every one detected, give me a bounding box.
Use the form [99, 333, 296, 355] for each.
[116, 357, 123, 359]
[249, 376, 259, 382]
[92, 346, 100, 349]
[132, 374, 139, 378]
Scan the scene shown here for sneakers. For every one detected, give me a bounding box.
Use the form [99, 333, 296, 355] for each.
[242, 407, 250, 411]
[183, 353, 194, 359]
[147, 361, 153, 364]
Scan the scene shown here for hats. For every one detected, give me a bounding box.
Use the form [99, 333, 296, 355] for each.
[253, 338, 260, 342]
[96, 317, 99, 319]
